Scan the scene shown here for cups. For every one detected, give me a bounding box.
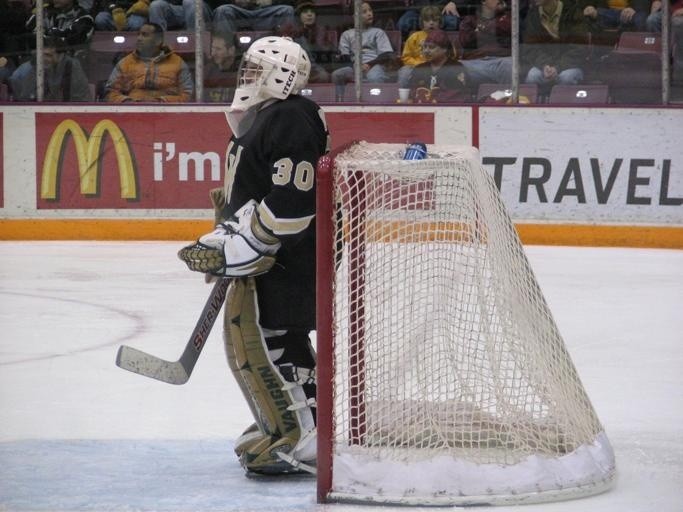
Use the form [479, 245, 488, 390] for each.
[398, 88, 410, 103]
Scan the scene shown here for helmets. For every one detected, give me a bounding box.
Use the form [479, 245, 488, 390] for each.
[230, 36, 311, 112]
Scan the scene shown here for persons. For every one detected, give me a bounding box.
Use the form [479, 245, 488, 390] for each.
[176, 35, 331, 478]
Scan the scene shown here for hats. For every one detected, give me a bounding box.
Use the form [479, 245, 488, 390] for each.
[425, 29, 449, 48]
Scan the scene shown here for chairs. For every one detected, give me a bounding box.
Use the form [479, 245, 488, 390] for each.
[1, 0, 683, 105]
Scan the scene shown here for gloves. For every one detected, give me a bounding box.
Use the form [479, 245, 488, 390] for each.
[178, 198, 281, 283]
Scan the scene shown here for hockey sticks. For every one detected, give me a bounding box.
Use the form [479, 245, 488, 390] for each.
[116, 272, 231, 384]
